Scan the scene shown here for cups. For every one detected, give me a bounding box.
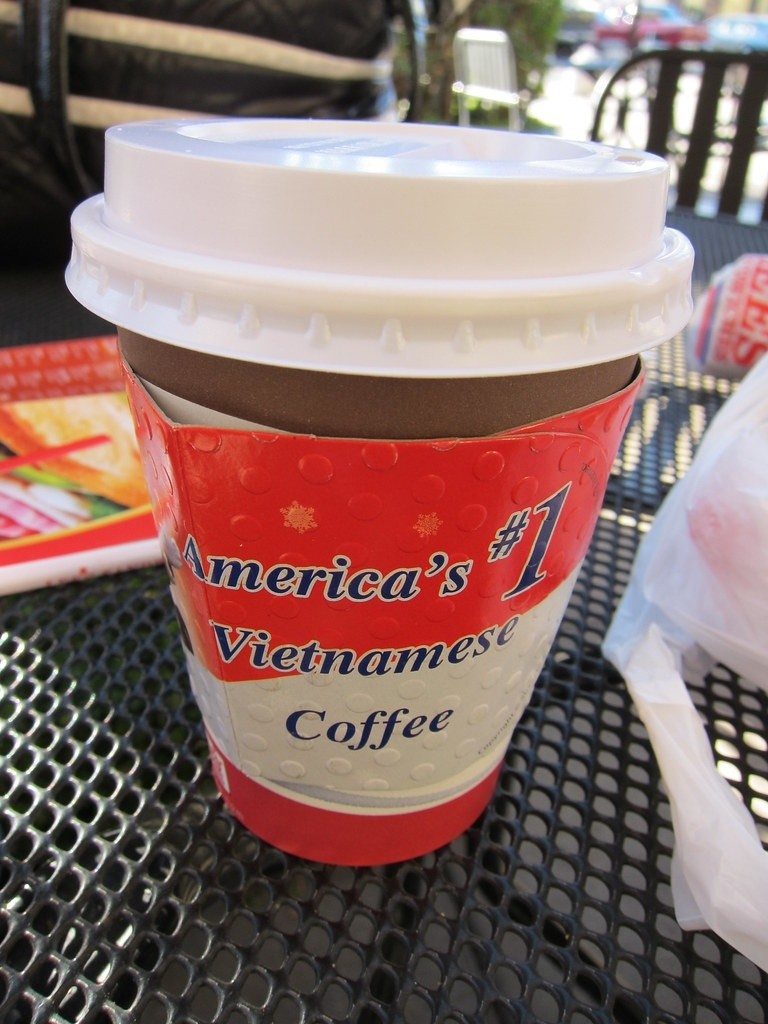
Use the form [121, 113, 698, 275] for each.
[64, 119, 691, 868]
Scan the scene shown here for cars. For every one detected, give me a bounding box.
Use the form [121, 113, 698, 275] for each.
[554, 0, 768, 74]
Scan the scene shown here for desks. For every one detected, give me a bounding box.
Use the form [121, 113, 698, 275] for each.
[0, 208, 768, 1024]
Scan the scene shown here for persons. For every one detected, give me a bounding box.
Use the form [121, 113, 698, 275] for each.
[611, 73, 633, 132]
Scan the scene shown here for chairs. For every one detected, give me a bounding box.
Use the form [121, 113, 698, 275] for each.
[587, 47, 767, 226]
